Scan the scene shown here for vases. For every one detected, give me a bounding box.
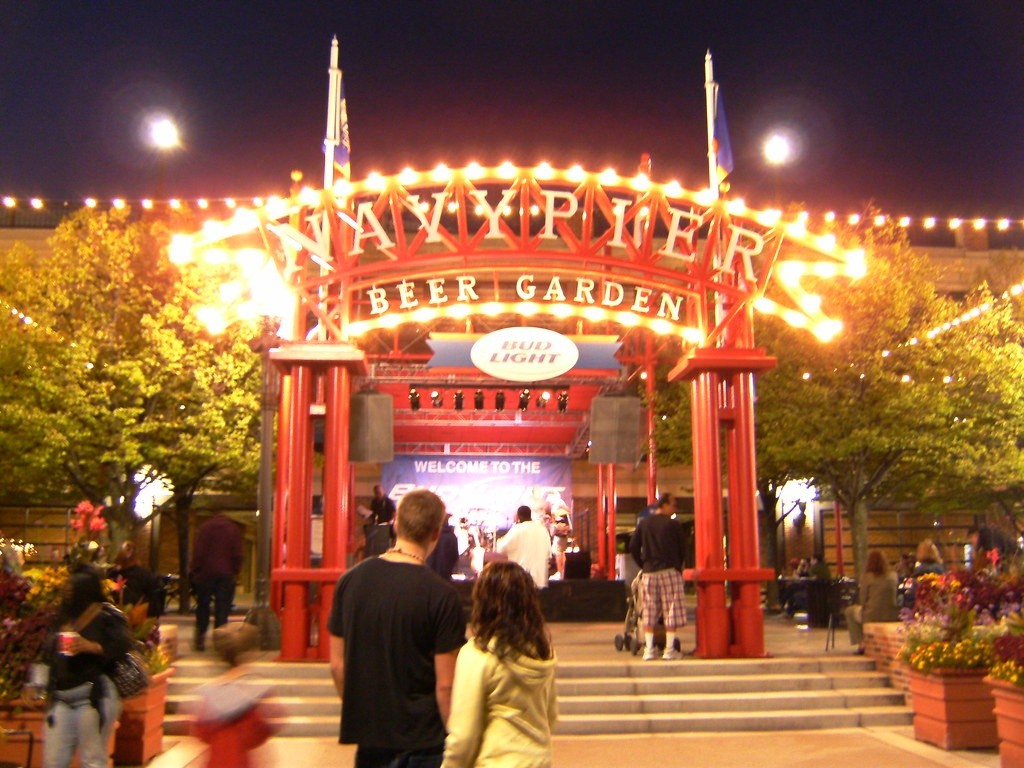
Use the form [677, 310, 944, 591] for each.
[980, 674, 1024, 768]
[0, 697, 119, 768]
[903, 659, 995, 752]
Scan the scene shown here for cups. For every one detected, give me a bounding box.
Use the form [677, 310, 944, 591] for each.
[60, 632, 80, 655]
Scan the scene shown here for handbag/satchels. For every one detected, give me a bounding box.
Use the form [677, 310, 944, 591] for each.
[112, 652, 149, 698]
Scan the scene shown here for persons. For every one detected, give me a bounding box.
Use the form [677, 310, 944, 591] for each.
[777, 552, 831, 619]
[437, 560, 560, 768]
[628, 491, 690, 661]
[358, 483, 573, 590]
[967, 525, 1024, 557]
[30, 562, 133, 768]
[845, 549, 900, 655]
[327, 491, 468, 768]
[898, 538, 944, 607]
[187, 499, 247, 652]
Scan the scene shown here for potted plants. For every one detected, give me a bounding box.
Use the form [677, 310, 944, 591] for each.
[117, 663, 179, 767]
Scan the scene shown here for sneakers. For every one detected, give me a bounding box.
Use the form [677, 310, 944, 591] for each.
[643, 647, 657, 660]
[662, 648, 683, 660]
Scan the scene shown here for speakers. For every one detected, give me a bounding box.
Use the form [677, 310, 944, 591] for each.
[565, 553, 591, 578]
[348, 391, 393, 463]
[589, 395, 641, 464]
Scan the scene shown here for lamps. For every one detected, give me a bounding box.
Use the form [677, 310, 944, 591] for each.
[517, 388, 532, 409]
[557, 390, 569, 413]
[429, 389, 444, 408]
[452, 388, 465, 411]
[408, 388, 421, 411]
[495, 389, 506, 410]
[473, 389, 486, 409]
[535, 390, 551, 410]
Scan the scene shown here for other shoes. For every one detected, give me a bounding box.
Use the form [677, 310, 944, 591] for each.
[852, 648, 863, 655]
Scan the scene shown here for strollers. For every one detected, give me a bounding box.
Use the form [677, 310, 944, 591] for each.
[615, 569, 681, 657]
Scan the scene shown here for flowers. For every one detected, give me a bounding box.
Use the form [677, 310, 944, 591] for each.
[902, 555, 1024, 685]
[0, 491, 166, 717]
